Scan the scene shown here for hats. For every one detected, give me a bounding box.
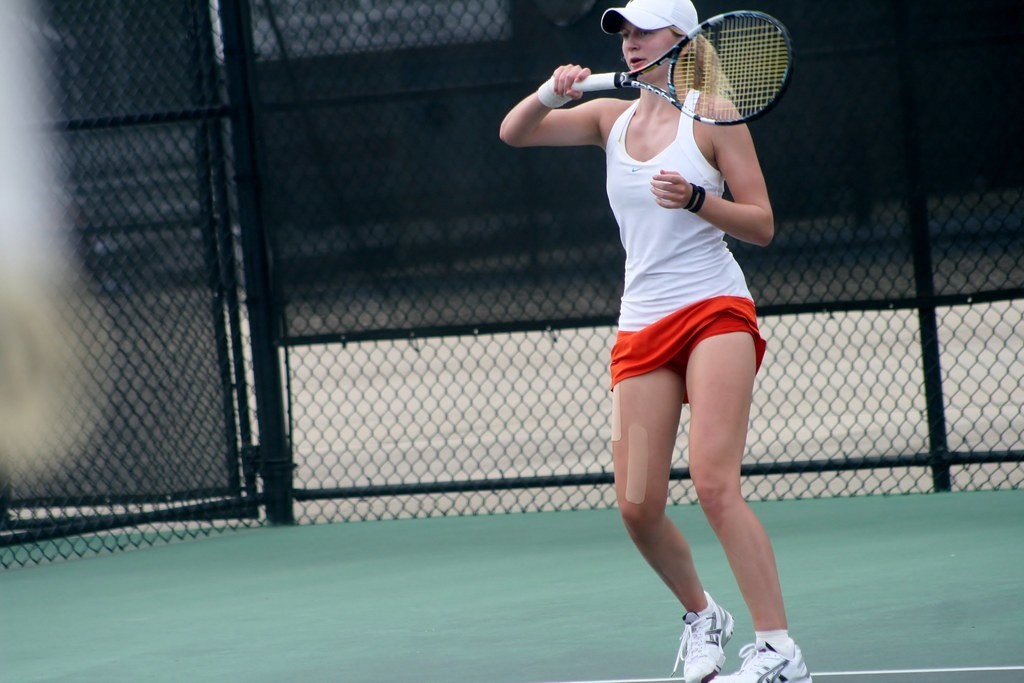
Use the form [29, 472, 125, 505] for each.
[601, 0, 698, 38]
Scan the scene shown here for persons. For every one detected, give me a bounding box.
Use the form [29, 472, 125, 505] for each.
[498, 0, 816, 682]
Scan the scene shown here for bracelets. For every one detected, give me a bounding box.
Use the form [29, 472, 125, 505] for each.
[688, 185, 706, 214]
[537, 75, 574, 109]
[683, 183, 698, 210]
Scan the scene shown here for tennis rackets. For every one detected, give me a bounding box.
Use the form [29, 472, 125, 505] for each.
[571, 8, 796, 127]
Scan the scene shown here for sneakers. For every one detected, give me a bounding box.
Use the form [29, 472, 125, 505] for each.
[735, 644, 812, 683]
[671, 590, 734, 683]
